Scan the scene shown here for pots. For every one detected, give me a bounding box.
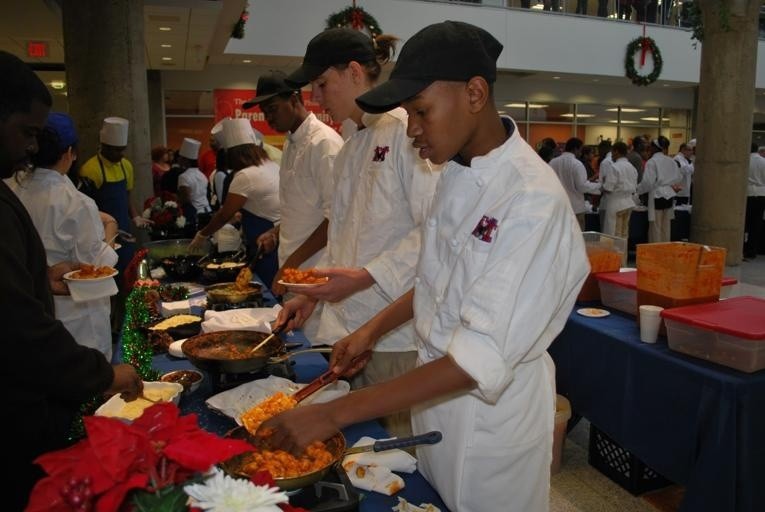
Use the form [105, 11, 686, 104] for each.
[161, 255, 197, 282]
[216, 422, 441, 491]
[200, 282, 263, 304]
[200, 259, 248, 282]
[181, 330, 335, 374]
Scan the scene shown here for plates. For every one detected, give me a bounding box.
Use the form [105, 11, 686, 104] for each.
[63, 268, 119, 282]
[277, 279, 323, 290]
[168, 339, 191, 359]
[579, 307, 609, 319]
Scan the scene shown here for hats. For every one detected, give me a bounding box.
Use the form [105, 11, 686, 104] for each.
[243, 70, 300, 109]
[212, 116, 256, 150]
[179, 138, 201, 160]
[41, 112, 79, 154]
[284, 29, 374, 89]
[101, 117, 129, 147]
[355, 21, 503, 114]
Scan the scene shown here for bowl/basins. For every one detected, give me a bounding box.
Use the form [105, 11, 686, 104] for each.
[160, 370, 205, 397]
[94, 378, 184, 425]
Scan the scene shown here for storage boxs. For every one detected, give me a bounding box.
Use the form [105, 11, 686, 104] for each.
[636, 238, 729, 325]
[660, 294, 765, 374]
[590, 267, 738, 323]
[572, 230, 628, 305]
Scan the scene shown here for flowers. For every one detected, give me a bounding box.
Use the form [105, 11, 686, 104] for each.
[24, 401, 315, 512]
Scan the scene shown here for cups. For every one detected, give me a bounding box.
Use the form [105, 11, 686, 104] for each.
[638, 305, 664, 345]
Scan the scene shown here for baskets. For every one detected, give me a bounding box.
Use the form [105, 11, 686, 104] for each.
[588, 424, 675, 496]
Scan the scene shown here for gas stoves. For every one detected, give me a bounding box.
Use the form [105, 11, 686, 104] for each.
[212, 351, 298, 397]
[288, 464, 360, 512]
[198, 294, 275, 317]
